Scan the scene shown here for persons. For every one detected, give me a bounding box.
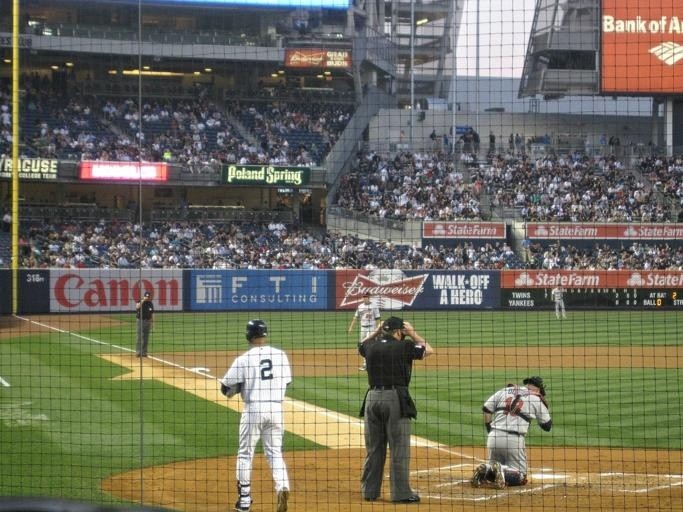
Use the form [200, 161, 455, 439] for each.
[551, 283, 567, 319]
[470, 376, 553, 489]
[220, 319, 292, 512]
[358, 315, 433, 503]
[135, 292, 155, 357]
[348, 292, 381, 372]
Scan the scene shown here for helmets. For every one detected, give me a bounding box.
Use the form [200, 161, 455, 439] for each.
[244, 318, 267, 341]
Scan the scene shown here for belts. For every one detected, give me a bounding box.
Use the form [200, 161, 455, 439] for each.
[369, 384, 396, 390]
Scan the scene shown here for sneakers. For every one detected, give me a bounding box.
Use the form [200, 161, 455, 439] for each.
[135, 352, 147, 358]
[470, 463, 490, 487]
[492, 461, 505, 488]
[276, 486, 289, 512]
[358, 365, 366, 371]
[362, 496, 376, 501]
[390, 493, 421, 503]
[234, 500, 250, 512]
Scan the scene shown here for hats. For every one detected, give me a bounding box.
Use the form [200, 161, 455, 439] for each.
[522, 375, 546, 395]
[380, 317, 404, 330]
[362, 291, 370, 297]
[143, 292, 150, 297]
